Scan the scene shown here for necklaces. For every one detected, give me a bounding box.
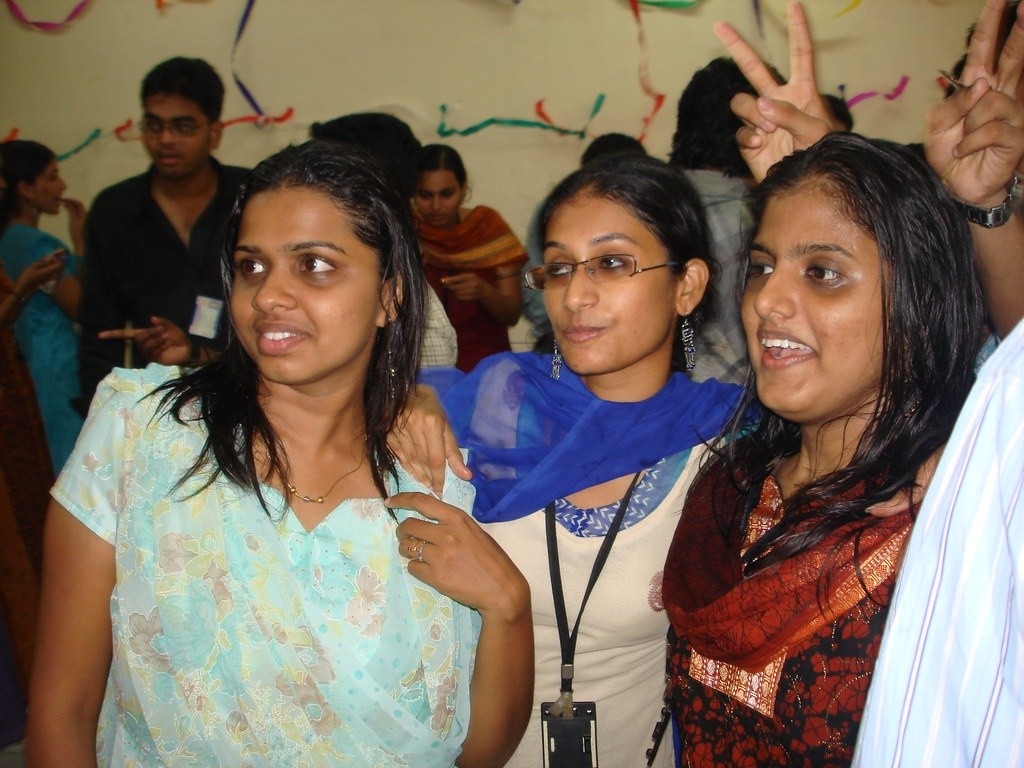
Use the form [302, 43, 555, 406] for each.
[253, 437, 367, 505]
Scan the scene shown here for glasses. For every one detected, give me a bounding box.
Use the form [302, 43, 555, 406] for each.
[135, 118, 213, 138]
[524, 253, 685, 291]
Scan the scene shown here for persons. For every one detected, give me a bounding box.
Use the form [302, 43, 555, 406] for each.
[661, 130, 987, 768]
[1, 3, 1022, 768]
[27, 145, 529, 768]
[390, 161, 758, 768]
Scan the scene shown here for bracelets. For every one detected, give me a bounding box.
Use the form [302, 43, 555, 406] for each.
[946, 179, 1019, 227]
